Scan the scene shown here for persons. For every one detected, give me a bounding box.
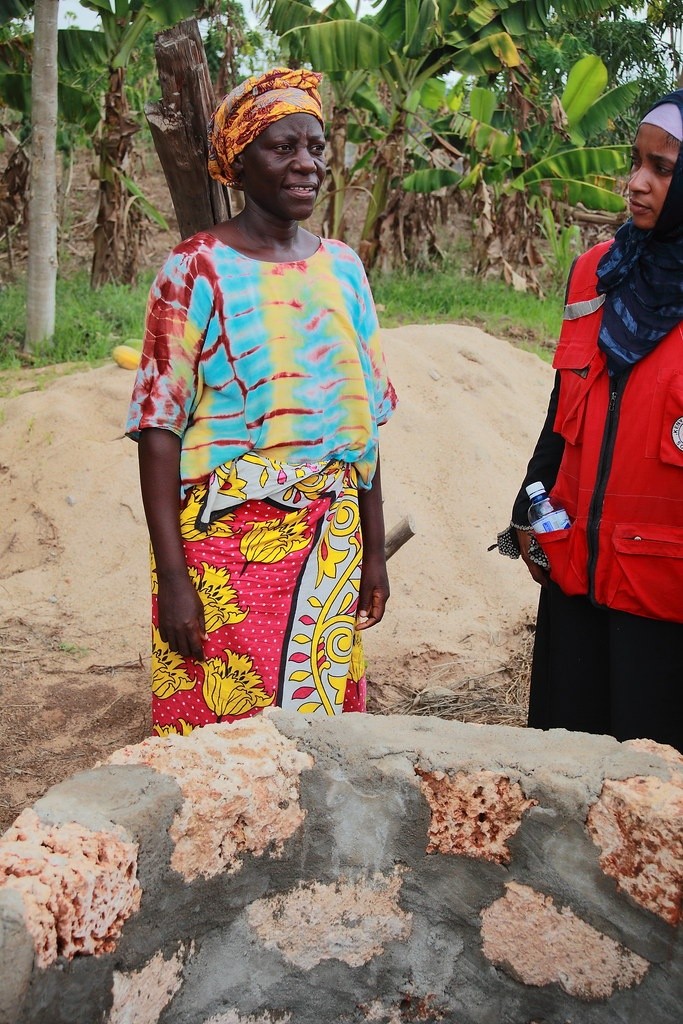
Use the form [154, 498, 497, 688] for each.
[123, 65, 400, 741]
[506, 85, 682, 752]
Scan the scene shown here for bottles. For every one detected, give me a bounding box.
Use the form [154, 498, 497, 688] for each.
[524, 480, 573, 535]
